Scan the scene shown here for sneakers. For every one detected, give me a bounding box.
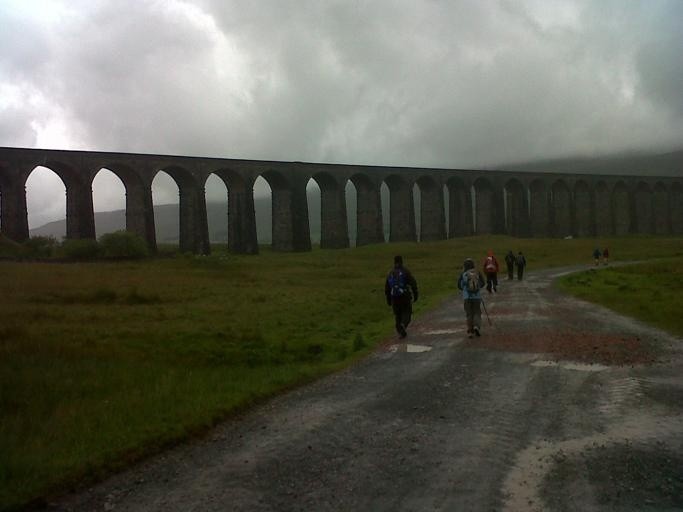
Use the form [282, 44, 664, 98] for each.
[467, 326, 482, 338]
[396, 323, 408, 339]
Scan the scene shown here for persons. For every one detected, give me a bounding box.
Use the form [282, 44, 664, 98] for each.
[592, 248, 601, 267]
[482, 247, 500, 294]
[516, 251, 527, 281]
[384, 254, 419, 339]
[603, 247, 609, 266]
[505, 250, 515, 282]
[457, 258, 486, 338]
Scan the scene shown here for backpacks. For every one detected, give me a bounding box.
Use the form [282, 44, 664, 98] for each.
[466, 269, 481, 293]
[388, 269, 408, 305]
